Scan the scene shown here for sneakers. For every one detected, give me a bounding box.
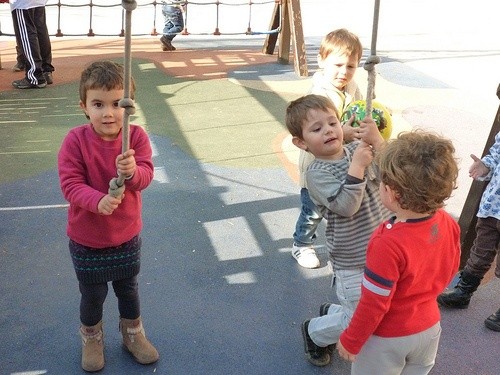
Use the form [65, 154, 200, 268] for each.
[12, 78, 46, 89]
[437, 289, 470, 308]
[13, 62, 24, 71]
[160, 36, 176, 51]
[291, 241, 320, 269]
[484, 308, 500, 332]
[302, 302, 338, 367]
[43, 72, 53, 83]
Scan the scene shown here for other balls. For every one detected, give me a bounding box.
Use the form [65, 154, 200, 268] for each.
[341, 100, 392, 144]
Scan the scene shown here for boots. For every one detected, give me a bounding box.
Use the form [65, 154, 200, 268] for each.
[119, 316, 159, 364]
[79, 320, 104, 371]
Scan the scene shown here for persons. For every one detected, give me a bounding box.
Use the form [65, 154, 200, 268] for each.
[292, 30, 363, 270]
[58, 61, 158, 372]
[160, 0, 188, 51]
[437, 82, 500, 331]
[286, 94, 395, 366]
[11, 0, 55, 89]
[337, 129, 461, 375]
